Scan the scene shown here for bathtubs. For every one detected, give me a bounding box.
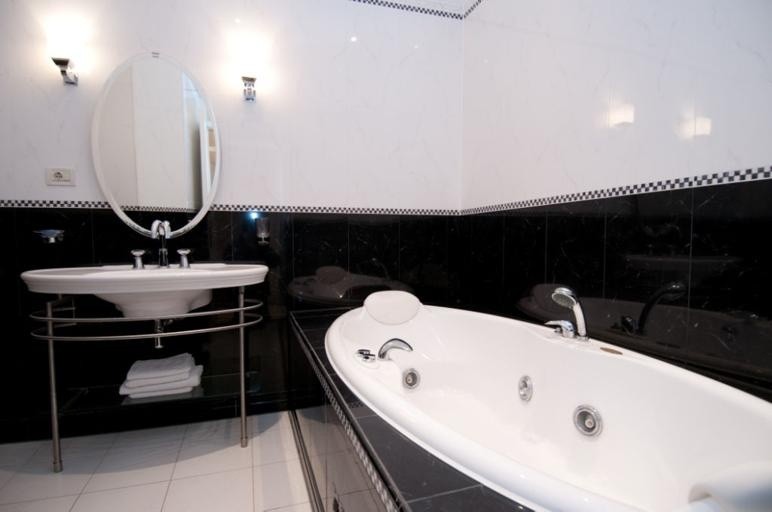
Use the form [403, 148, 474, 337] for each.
[324, 291, 772, 512]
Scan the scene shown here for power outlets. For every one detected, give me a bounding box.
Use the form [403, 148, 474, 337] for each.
[46, 167, 74, 186]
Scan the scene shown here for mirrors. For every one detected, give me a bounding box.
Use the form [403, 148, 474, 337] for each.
[89, 50, 222, 242]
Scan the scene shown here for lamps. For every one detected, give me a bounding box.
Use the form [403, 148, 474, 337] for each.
[52, 57, 79, 87]
[240, 75, 256, 103]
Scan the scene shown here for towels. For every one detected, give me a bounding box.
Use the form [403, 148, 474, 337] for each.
[119, 353, 204, 400]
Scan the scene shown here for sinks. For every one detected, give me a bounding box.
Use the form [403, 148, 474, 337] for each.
[21, 263, 269, 318]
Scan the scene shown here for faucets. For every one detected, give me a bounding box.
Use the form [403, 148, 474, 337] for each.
[151, 220, 172, 266]
[378, 338, 413, 358]
